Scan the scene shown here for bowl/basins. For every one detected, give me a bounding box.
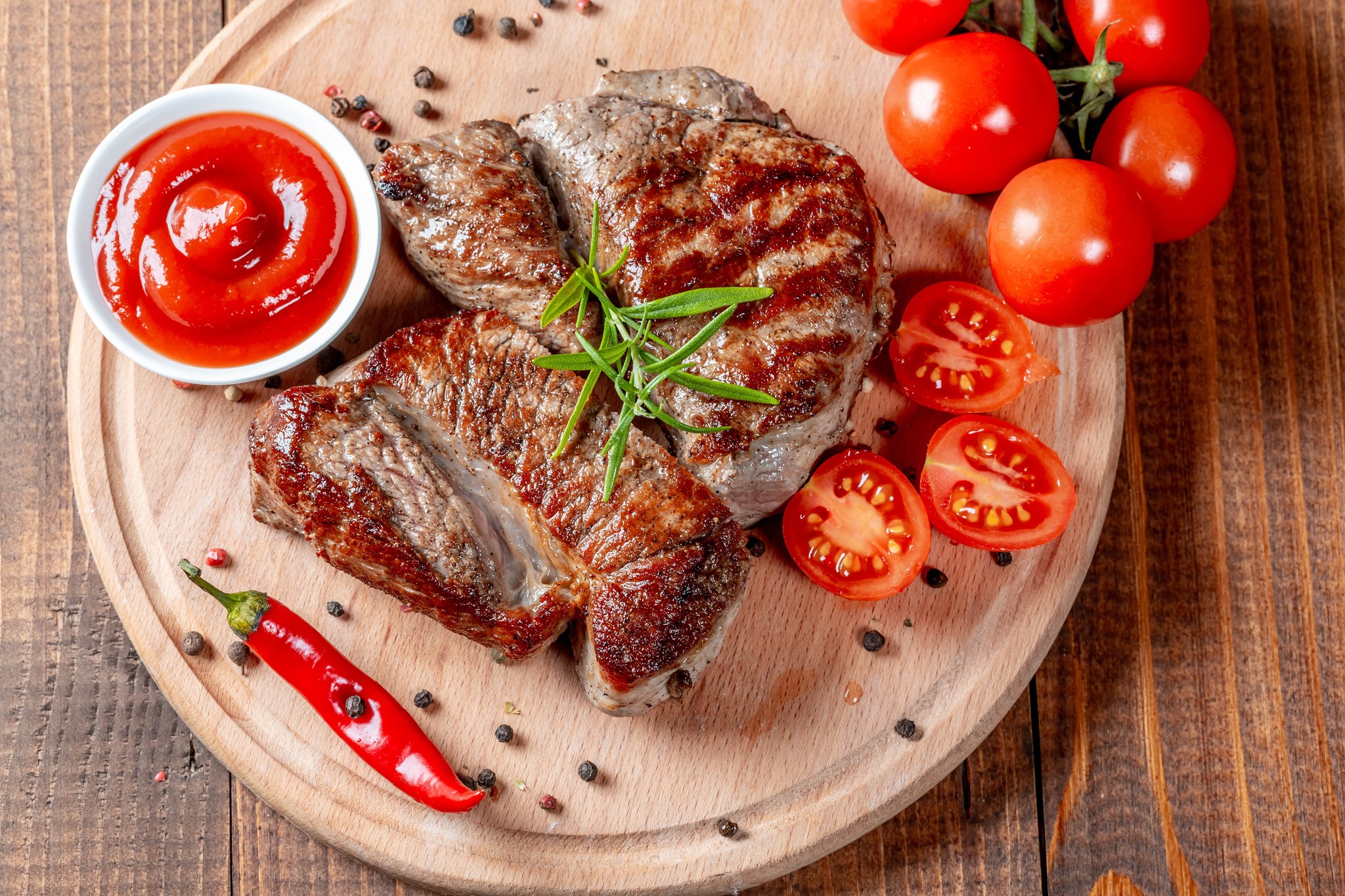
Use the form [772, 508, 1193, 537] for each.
[66, 84, 383, 392]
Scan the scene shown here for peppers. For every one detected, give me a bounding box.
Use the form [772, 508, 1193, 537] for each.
[176, 557, 486, 814]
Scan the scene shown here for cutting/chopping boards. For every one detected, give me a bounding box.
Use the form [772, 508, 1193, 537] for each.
[68, 1, 1130, 894]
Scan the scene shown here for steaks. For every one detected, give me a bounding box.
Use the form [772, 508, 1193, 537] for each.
[248, 62, 904, 729]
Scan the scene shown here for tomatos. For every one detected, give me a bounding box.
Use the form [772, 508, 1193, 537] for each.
[774, 3, 1239, 605]
